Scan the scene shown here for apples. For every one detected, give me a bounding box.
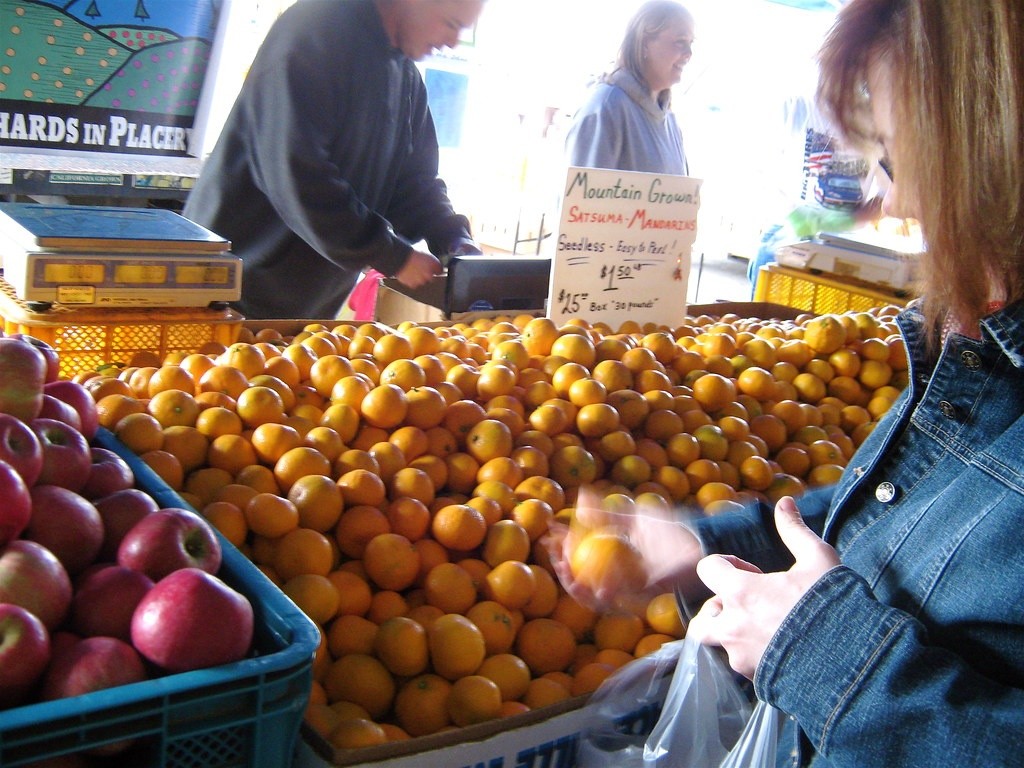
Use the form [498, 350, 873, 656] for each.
[0, 329, 253, 723]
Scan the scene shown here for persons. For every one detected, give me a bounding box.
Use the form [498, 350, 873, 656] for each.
[564, 0, 696, 176]
[546, 0, 1024, 768]
[181, 0, 488, 320]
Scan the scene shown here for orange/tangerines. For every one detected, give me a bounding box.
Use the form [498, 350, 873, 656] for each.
[74, 305, 907, 750]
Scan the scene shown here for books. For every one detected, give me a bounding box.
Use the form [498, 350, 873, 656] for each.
[819, 216, 924, 258]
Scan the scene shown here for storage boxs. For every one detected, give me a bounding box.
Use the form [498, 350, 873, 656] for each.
[754, 262, 914, 315]
[301, 677, 672, 768]
[0, 276, 245, 383]
[373, 275, 546, 324]
[687, 301, 815, 320]
[0, 424, 322, 768]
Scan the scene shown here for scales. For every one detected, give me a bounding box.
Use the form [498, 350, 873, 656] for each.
[774, 214, 934, 298]
[0, 200, 244, 311]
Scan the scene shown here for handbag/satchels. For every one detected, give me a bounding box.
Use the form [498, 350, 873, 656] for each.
[577, 630, 782, 768]
